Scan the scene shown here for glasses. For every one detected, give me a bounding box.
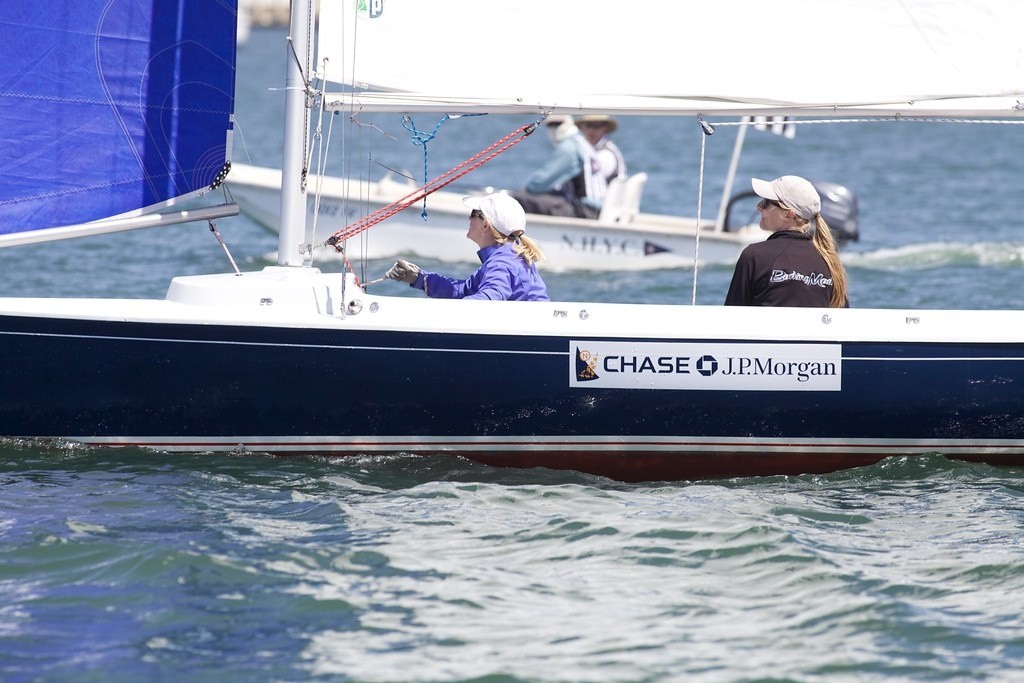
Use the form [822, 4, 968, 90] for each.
[471, 209, 484, 220]
[761, 198, 781, 208]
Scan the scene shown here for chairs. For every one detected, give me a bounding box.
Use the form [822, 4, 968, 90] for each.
[624, 171, 648, 223]
[598, 175, 628, 222]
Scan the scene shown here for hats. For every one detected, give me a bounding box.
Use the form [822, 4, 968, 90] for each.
[575, 115, 617, 134]
[751, 175, 821, 220]
[463, 193, 526, 238]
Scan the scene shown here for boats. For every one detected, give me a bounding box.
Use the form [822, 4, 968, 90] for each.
[220, 163, 860, 273]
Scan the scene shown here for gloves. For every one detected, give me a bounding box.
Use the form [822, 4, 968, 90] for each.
[385, 257, 421, 284]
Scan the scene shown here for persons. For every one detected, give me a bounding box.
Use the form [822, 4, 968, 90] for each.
[507, 113, 607, 219]
[724, 175, 849, 308]
[385, 192, 551, 302]
[575, 114, 626, 182]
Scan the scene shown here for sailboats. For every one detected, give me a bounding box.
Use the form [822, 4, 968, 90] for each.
[0, 1, 1024, 485]
